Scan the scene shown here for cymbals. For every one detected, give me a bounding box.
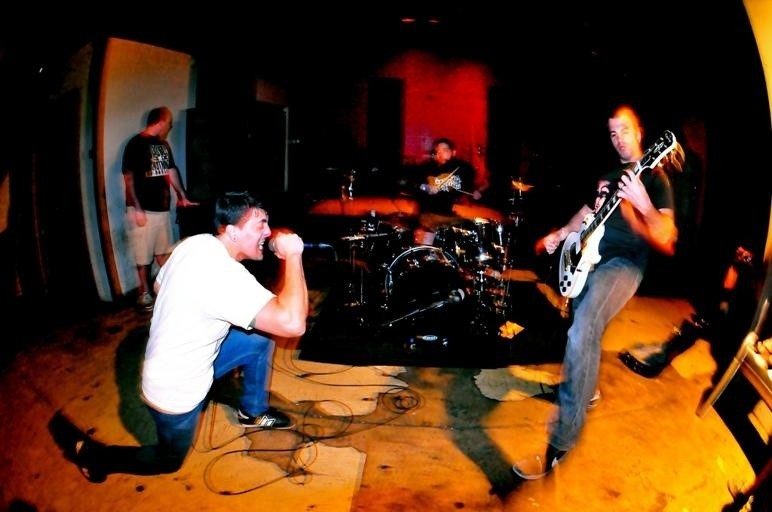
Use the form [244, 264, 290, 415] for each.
[509, 175, 532, 192]
[328, 167, 379, 174]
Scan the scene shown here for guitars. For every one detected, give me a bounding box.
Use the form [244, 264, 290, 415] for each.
[557, 130, 678, 299]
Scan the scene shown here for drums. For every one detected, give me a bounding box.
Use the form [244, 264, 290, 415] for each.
[466, 218, 505, 246]
[342, 213, 410, 274]
[433, 224, 479, 263]
[384, 246, 475, 354]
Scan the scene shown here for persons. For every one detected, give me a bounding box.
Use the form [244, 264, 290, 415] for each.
[621, 238, 766, 411]
[122, 106, 201, 311]
[511, 101, 680, 479]
[73, 192, 312, 485]
[409, 138, 484, 227]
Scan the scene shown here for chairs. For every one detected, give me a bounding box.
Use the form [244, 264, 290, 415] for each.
[695, 271, 772, 497]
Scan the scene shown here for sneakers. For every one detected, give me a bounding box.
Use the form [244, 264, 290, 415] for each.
[66, 436, 107, 484]
[237, 406, 297, 431]
[586, 387, 603, 411]
[512, 443, 571, 481]
[135, 292, 154, 312]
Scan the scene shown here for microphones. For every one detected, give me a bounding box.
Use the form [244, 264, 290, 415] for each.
[266, 236, 332, 252]
[447, 286, 465, 304]
